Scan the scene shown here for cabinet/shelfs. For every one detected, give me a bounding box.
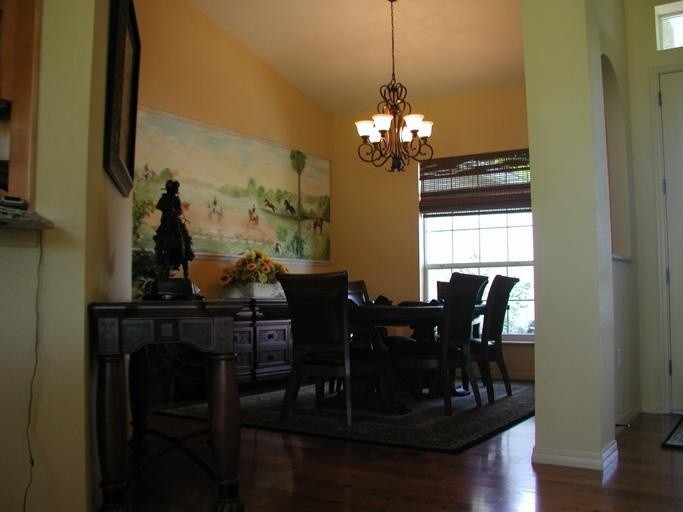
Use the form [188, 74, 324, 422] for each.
[253, 297, 292, 391]
[233, 297, 253, 395]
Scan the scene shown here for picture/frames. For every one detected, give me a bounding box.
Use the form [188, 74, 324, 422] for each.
[133, 104, 334, 265]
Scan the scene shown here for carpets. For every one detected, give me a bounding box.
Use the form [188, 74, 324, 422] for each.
[150, 380, 535, 454]
[660, 415, 683, 450]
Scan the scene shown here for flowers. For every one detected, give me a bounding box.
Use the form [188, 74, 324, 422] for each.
[217, 250, 289, 284]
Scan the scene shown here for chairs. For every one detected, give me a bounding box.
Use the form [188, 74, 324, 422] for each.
[397, 272, 489, 416]
[103, 1, 142, 200]
[276, 270, 366, 427]
[436, 280, 481, 339]
[346, 279, 370, 310]
[450, 274, 521, 404]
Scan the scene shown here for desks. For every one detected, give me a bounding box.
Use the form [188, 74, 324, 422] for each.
[355, 302, 510, 398]
[89, 296, 247, 512]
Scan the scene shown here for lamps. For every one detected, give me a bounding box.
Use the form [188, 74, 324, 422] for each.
[354, 0, 434, 173]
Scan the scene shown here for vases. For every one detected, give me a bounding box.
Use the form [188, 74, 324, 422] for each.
[239, 284, 274, 299]
